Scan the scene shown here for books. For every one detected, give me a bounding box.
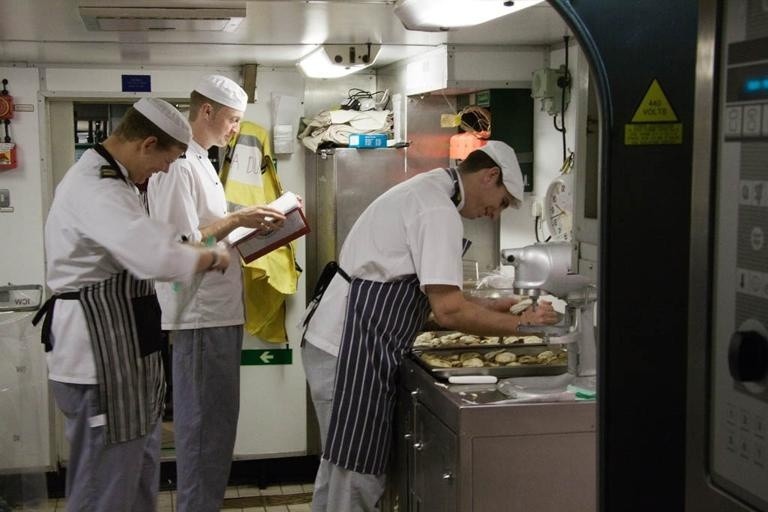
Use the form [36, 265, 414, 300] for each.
[226, 190, 310, 264]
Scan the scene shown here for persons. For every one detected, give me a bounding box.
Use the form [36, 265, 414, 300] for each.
[146, 74, 305, 510]
[30, 92, 229, 511]
[298, 138, 562, 512]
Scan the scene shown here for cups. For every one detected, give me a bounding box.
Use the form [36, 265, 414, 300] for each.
[461, 259, 481, 298]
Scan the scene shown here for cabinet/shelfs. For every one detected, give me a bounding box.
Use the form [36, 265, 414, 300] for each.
[382, 363, 474, 512]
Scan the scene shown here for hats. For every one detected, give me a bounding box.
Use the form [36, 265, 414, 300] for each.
[194, 74, 248, 111]
[477, 139, 524, 209]
[133, 95, 192, 146]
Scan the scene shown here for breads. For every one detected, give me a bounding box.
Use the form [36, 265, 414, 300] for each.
[411, 300, 571, 370]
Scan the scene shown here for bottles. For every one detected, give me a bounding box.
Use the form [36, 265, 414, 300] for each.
[95, 120, 102, 142]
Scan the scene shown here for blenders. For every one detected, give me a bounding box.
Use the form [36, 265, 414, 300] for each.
[496, 243, 601, 403]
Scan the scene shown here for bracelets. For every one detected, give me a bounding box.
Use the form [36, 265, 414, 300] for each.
[517, 312, 524, 332]
[209, 248, 220, 272]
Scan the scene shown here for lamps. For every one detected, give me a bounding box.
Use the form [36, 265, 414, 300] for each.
[296, 44, 383, 79]
[391, 0, 544, 32]
